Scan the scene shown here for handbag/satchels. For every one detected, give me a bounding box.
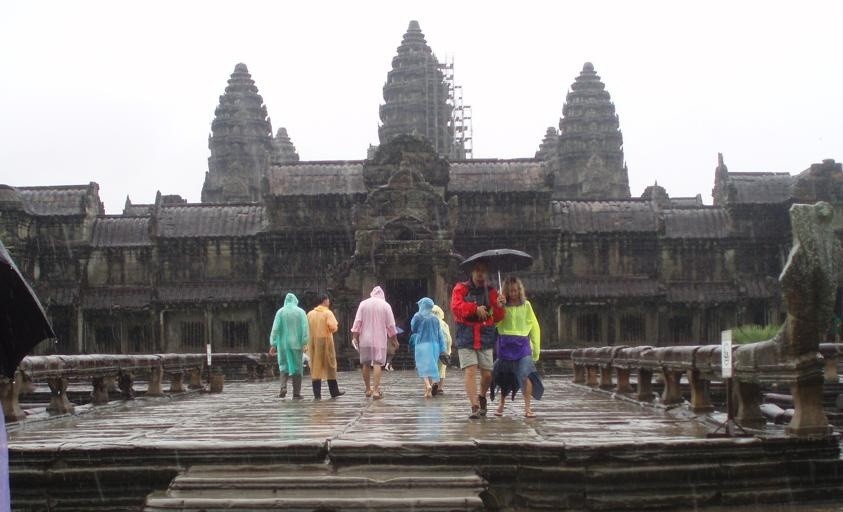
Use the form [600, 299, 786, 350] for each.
[464, 290, 485, 322]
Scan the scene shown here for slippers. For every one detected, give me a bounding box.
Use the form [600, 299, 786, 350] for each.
[494, 410, 503, 416]
[366, 391, 383, 399]
[524, 412, 536, 417]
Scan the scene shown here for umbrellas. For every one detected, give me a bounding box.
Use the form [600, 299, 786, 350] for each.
[393, 325, 404, 335]
[459, 248, 533, 296]
[0, 236, 55, 386]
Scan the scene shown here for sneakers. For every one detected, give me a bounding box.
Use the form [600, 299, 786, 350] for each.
[469, 405, 480, 419]
[480, 397, 487, 416]
[425, 383, 443, 397]
[279, 388, 346, 399]
[385, 367, 394, 371]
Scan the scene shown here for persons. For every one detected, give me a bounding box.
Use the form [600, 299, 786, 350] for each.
[384, 334, 396, 372]
[431, 304, 452, 392]
[489, 274, 545, 418]
[306, 293, 345, 399]
[267, 293, 309, 400]
[407, 297, 449, 397]
[349, 285, 399, 399]
[448, 260, 506, 419]
